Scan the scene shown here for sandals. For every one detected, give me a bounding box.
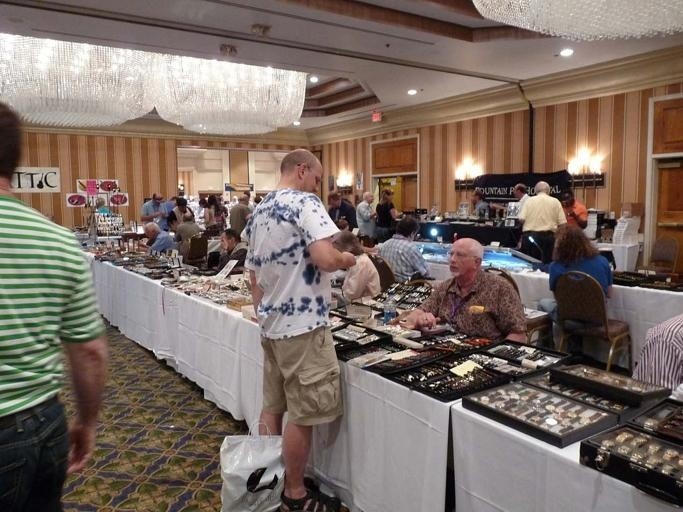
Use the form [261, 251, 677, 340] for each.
[280, 489, 349, 512]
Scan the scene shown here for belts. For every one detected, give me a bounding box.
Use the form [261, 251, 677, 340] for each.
[0, 395, 60, 429]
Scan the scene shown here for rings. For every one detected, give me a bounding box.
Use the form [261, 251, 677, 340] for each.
[422, 315, 428, 321]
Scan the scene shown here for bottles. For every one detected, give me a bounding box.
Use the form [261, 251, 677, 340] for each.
[383, 290, 397, 326]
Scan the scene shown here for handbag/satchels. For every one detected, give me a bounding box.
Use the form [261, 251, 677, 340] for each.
[219, 420, 284, 512]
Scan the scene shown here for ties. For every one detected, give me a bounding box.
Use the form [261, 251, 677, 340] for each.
[449, 295, 466, 322]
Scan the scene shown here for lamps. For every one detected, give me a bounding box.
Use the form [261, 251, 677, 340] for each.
[472, 0, 683, 43]
[0, 31, 155, 128]
[150, 52, 307, 135]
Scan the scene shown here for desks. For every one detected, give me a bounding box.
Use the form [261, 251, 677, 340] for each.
[510, 267, 683, 370]
[451, 401, 683, 512]
[244, 316, 451, 512]
[82, 243, 162, 360]
[426, 262, 455, 281]
[162, 260, 244, 421]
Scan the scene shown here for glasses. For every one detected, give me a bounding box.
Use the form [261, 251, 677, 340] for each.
[446, 250, 479, 260]
[297, 161, 322, 185]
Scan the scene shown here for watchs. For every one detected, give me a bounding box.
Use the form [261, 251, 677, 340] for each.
[471, 382, 683, 478]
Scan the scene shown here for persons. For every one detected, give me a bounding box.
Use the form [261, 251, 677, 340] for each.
[1, 102, 109, 512]
[630, 313, 683, 393]
[537, 224, 614, 350]
[137, 188, 264, 277]
[244, 148, 350, 512]
[322, 179, 588, 302]
[415, 238, 528, 345]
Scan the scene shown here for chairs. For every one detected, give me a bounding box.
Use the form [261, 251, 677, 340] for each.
[555, 270, 632, 377]
[376, 255, 432, 290]
[484, 268, 553, 346]
[186, 234, 208, 270]
